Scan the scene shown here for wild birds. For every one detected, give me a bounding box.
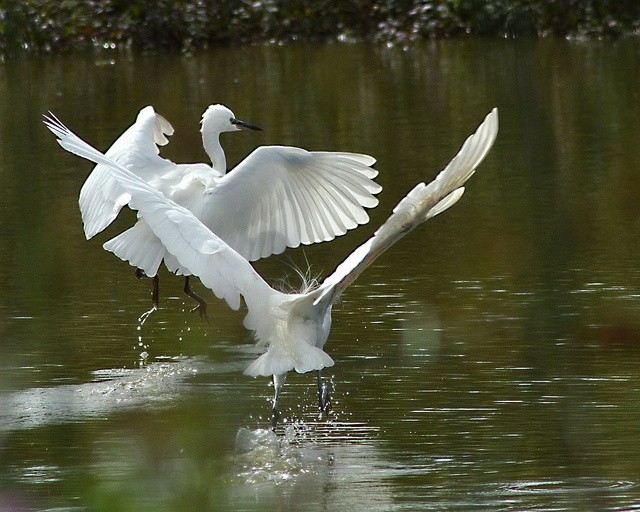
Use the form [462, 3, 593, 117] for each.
[79, 103, 383, 329]
[42, 107, 500, 433]
